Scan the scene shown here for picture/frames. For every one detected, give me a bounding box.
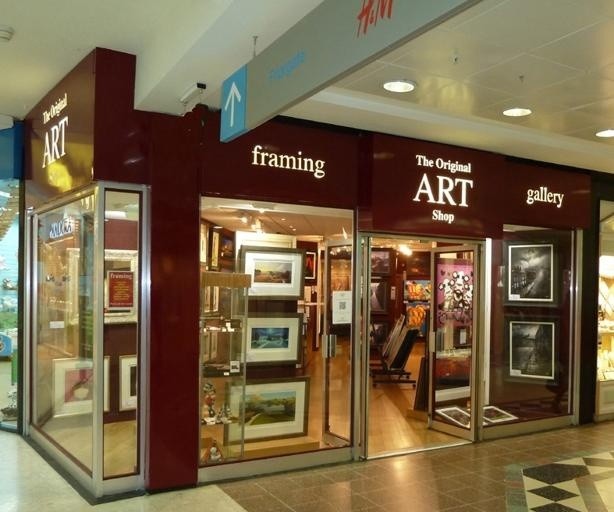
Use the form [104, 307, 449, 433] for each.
[434, 405, 493, 430]
[236, 312, 305, 371]
[237, 245, 306, 300]
[502, 238, 565, 307]
[223, 382, 310, 445]
[368, 247, 395, 315]
[200, 220, 223, 369]
[432, 257, 477, 332]
[51, 354, 111, 420]
[483, 406, 524, 421]
[504, 315, 561, 387]
[64, 249, 140, 326]
[116, 355, 139, 413]
